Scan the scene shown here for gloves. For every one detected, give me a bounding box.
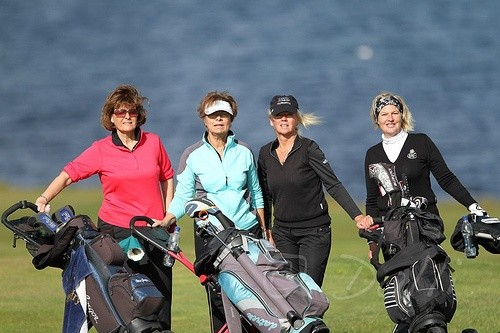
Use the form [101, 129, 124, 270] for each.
[468, 203, 490, 217]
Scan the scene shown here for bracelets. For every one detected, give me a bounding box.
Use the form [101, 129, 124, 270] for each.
[41, 195, 48, 203]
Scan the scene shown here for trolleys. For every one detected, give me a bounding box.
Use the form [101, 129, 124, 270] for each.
[130, 197, 330, 333]
[359, 162, 478, 333]
[1, 200, 163, 333]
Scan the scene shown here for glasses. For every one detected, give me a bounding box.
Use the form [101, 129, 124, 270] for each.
[112, 108, 138, 118]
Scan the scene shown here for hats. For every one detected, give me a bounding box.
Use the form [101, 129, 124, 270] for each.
[204, 100, 234, 117]
[270, 95, 298, 115]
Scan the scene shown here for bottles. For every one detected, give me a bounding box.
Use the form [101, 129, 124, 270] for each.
[461, 215, 476, 257]
[163, 226, 181, 267]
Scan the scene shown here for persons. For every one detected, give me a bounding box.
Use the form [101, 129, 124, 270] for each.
[255, 95, 374, 290]
[146, 89, 266, 333]
[365, 91, 490, 262]
[36, 85, 174, 333]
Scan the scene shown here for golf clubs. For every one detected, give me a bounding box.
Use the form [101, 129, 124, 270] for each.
[184, 197, 234, 237]
[26, 205, 75, 233]
[369, 162, 428, 211]
